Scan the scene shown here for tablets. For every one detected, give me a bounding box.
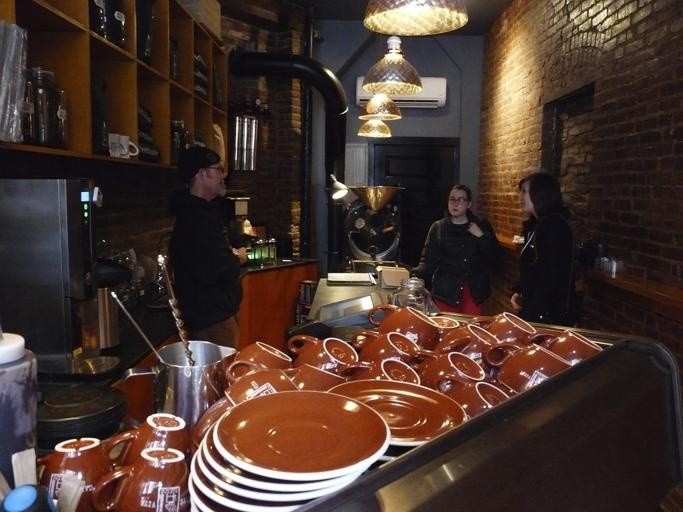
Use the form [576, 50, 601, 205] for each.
[318, 295, 374, 322]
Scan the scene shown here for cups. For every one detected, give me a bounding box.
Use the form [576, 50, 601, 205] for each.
[108, 133, 127, 157]
[120, 135, 140, 159]
[42, 303, 605, 508]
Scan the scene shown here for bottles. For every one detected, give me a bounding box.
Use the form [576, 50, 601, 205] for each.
[23, 66, 69, 149]
[170, 119, 192, 165]
[0, 332, 38, 485]
[389, 276, 433, 315]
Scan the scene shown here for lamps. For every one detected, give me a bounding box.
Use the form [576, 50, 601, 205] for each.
[356, 0, 468, 138]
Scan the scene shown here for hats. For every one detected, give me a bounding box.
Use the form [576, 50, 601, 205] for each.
[170, 146, 221, 184]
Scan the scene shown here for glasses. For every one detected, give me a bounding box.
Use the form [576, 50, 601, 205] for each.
[203, 165, 224, 174]
[447, 195, 468, 203]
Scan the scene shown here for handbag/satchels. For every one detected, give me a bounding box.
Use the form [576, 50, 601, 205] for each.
[519, 255, 576, 315]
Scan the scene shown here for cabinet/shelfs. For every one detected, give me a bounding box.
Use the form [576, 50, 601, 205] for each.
[0, 0, 228, 179]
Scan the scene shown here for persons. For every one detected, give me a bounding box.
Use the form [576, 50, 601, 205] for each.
[168, 145, 246, 349]
[509, 172, 589, 326]
[412, 185, 505, 315]
